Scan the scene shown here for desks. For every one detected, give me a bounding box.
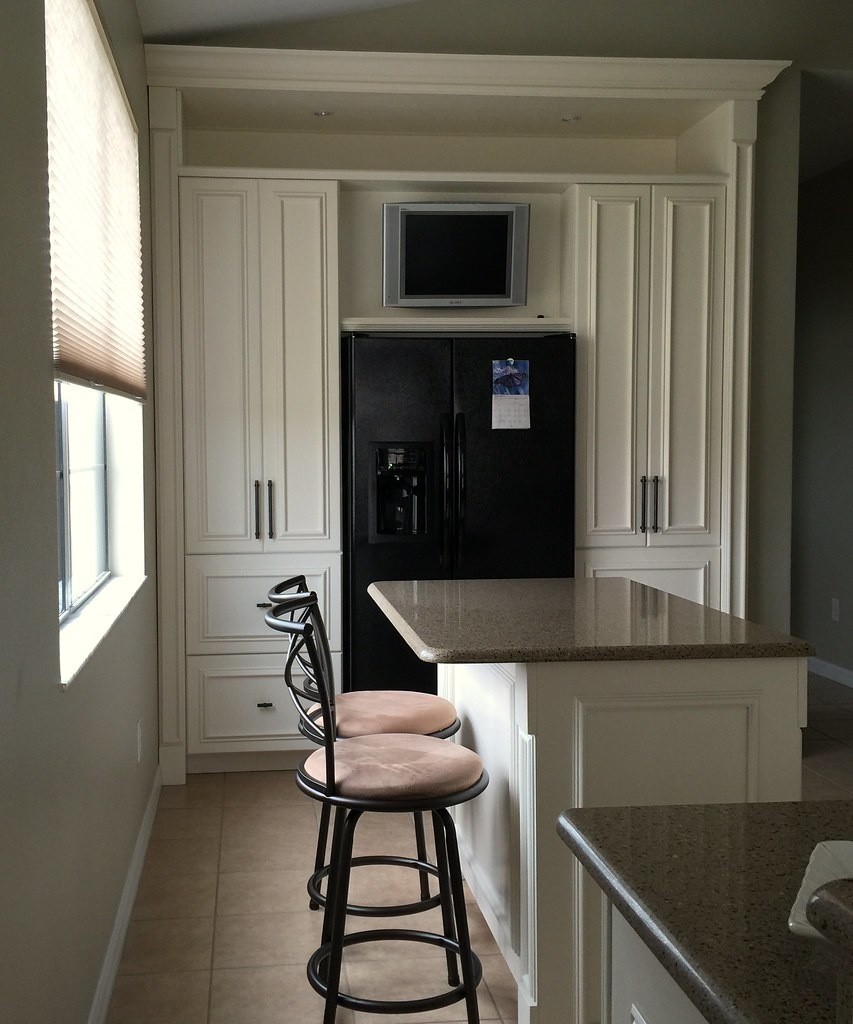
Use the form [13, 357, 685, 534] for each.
[363, 579, 852, 1024]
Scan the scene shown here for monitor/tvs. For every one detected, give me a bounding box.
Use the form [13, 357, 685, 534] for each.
[381, 202, 530, 309]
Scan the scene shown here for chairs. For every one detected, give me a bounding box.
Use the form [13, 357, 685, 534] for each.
[263, 575, 489, 1023]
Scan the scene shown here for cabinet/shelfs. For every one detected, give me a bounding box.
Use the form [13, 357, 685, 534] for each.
[173, 172, 746, 769]
